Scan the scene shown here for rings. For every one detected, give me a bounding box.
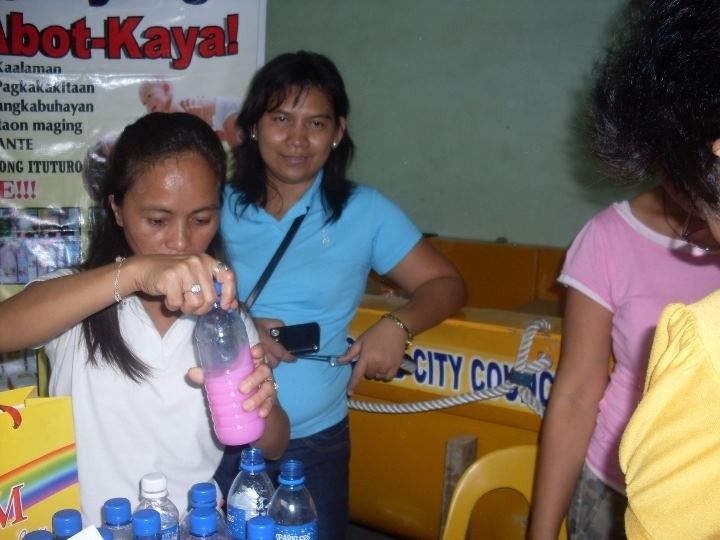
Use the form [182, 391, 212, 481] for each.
[259, 379, 280, 393]
[213, 262, 230, 277]
[182, 284, 202, 296]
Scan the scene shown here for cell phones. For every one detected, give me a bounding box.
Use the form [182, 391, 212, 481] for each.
[267, 322, 320, 354]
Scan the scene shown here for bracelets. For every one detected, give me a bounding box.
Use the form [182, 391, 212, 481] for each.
[381, 314, 412, 349]
[114, 257, 125, 302]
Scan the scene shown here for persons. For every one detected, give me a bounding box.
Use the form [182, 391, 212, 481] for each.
[0, 113, 290, 527]
[81, 75, 243, 196]
[618, 107, 720, 540]
[528, 0, 720, 540]
[220, 50, 468, 540]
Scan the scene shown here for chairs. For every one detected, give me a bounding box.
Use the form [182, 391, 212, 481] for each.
[443, 444, 568, 540]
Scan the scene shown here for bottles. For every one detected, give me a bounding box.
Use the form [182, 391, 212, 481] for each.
[193, 280, 265, 446]
[23, 445, 319, 540]
